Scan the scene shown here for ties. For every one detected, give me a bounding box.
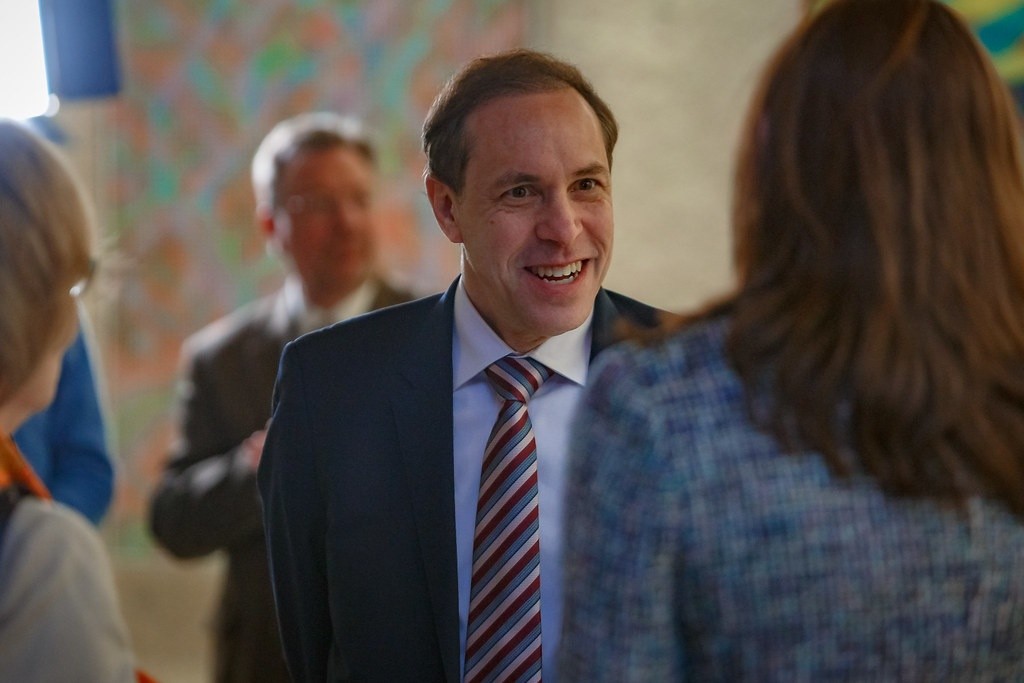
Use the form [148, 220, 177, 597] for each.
[464, 352, 555, 683]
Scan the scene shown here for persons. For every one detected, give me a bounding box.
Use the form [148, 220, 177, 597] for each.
[145, 113, 416, 683]
[0, 116, 140, 683]
[10, 314, 113, 523]
[257, 49, 673, 683]
[551, 2, 1024, 683]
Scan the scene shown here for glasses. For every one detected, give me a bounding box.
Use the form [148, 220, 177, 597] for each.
[69, 259, 93, 297]
